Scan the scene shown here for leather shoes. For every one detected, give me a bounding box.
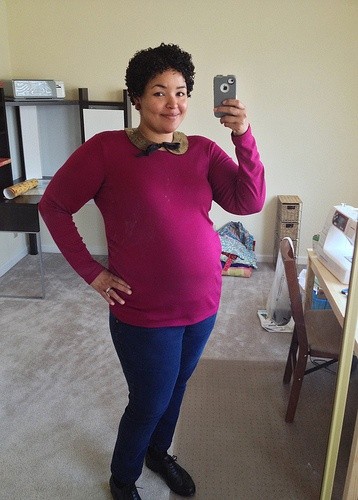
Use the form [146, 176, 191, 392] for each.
[109, 474, 141, 500]
[146, 453, 196, 496]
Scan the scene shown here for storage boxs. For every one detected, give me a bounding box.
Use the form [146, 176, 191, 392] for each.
[11, 79, 66, 100]
[272, 195, 304, 265]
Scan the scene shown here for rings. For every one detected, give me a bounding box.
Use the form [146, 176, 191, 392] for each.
[104, 286, 112, 294]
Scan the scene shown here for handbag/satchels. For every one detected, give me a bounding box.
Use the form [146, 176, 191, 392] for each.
[265, 236, 296, 326]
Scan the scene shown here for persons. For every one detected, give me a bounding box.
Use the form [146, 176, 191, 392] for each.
[39, 41, 266, 500]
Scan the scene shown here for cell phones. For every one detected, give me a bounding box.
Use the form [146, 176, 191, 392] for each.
[214, 75, 236, 117]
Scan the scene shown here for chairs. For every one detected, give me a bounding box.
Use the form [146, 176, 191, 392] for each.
[278, 236, 339, 423]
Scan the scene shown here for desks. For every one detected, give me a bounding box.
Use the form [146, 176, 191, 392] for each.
[0, 181, 48, 303]
[299, 246, 358, 361]
